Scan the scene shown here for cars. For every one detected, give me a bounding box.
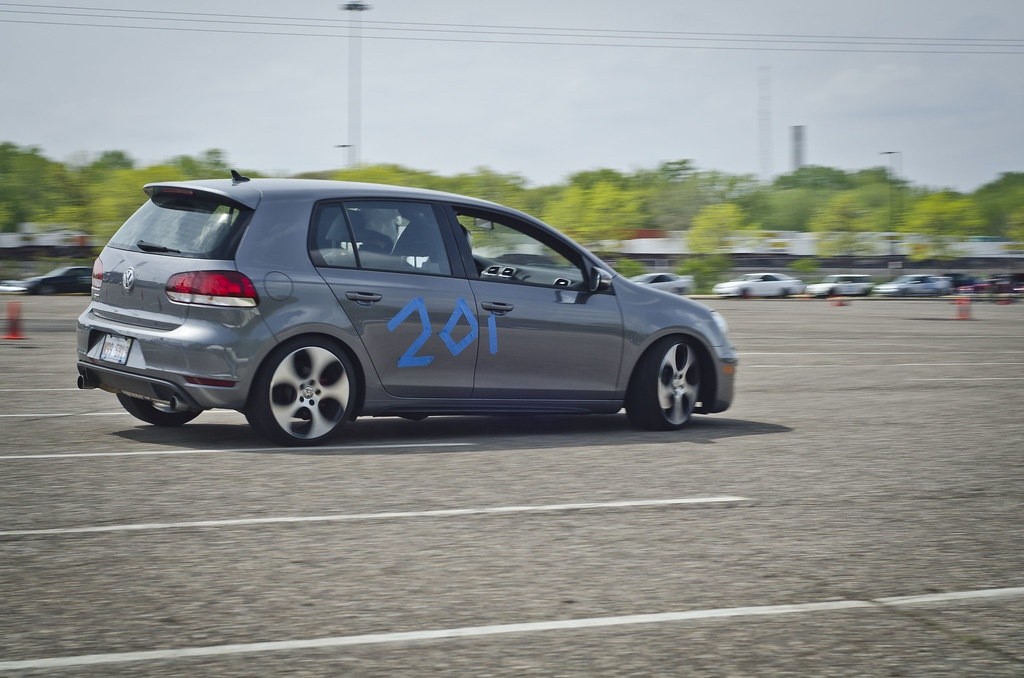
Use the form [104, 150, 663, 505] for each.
[74, 167, 738, 448]
[940, 271, 1024, 299]
[28, 265, 96, 297]
[871, 274, 946, 299]
[629, 271, 696, 295]
[712, 272, 805, 300]
[805, 274, 873, 299]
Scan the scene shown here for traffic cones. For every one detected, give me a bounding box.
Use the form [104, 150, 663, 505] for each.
[0, 300, 29, 341]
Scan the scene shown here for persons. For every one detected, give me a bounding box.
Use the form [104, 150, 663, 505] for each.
[359, 207, 402, 257]
[428, 222, 473, 278]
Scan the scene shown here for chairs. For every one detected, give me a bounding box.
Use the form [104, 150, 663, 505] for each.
[425, 221, 474, 275]
[317, 209, 366, 267]
